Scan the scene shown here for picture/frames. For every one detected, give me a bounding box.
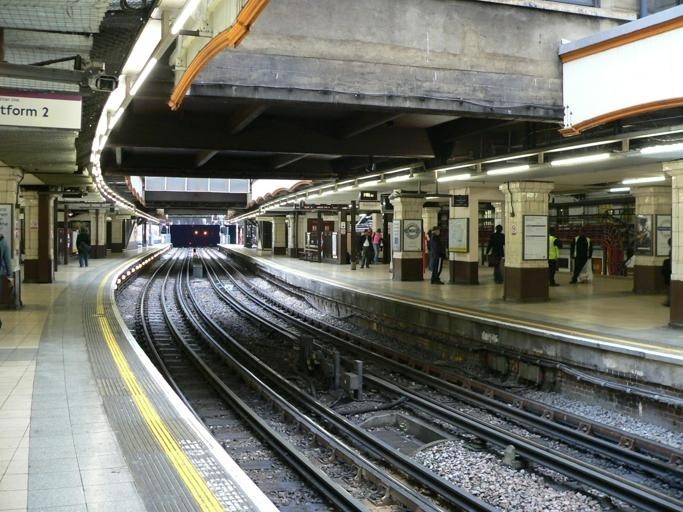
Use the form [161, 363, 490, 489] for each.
[522, 215, 549, 261]
[448, 218, 469, 253]
[655, 214, 672, 257]
[403, 219, 423, 252]
[392, 219, 401, 252]
[635, 214, 653, 256]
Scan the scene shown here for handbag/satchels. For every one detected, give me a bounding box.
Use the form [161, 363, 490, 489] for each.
[577, 258, 593, 283]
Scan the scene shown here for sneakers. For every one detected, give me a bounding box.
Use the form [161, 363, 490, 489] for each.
[360, 259, 378, 269]
[430, 278, 445, 285]
[80, 263, 89, 267]
[550, 279, 578, 287]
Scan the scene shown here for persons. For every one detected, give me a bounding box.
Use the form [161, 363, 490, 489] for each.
[75, 224, 90, 268]
[485, 224, 505, 284]
[0, 233, 14, 330]
[568, 228, 593, 283]
[359, 228, 373, 269]
[425, 229, 433, 273]
[372, 228, 383, 263]
[428, 226, 447, 284]
[549, 231, 561, 286]
